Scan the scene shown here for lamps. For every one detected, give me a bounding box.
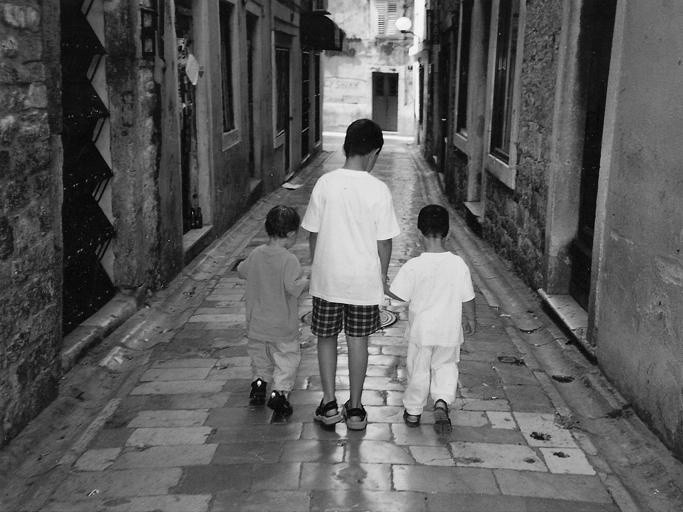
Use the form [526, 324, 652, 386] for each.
[396, 17, 420, 43]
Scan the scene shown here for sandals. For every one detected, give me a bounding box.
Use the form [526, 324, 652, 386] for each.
[403, 399, 452, 435]
[314, 398, 368, 430]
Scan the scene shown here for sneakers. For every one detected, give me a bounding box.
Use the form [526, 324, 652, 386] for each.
[249, 378, 293, 416]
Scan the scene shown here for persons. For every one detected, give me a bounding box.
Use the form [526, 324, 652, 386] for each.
[383, 203, 478, 434]
[235, 203, 310, 418]
[299, 118, 402, 430]
